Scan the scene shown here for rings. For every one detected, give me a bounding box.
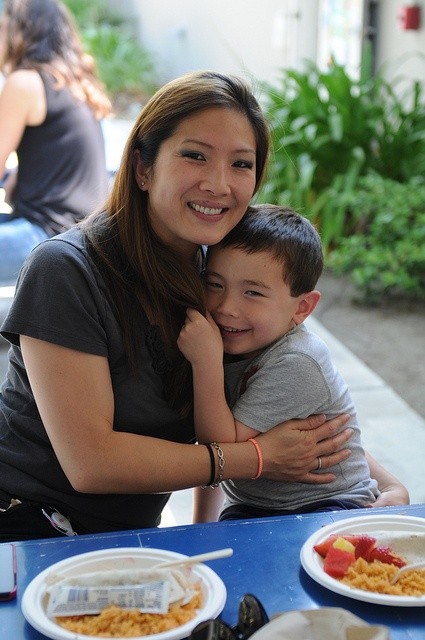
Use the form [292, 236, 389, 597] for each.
[315, 457, 321, 471]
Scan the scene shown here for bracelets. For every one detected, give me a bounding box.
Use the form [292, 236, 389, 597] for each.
[200, 443, 216, 490]
[249, 438, 264, 480]
[210, 441, 224, 489]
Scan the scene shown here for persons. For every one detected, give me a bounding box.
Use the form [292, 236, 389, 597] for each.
[176, 203, 381, 522]
[0, 0, 113, 290]
[0, 70, 411, 544]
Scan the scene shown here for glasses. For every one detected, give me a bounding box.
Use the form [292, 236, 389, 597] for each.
[191, 593, 269, 639]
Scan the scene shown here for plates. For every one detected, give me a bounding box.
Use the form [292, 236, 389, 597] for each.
[21, 547, 227, 639]
[300, 515, 425, 606]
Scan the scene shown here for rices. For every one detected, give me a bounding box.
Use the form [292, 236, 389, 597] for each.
[55, 585, 201, 637]
[340, 556, 425, 596]
